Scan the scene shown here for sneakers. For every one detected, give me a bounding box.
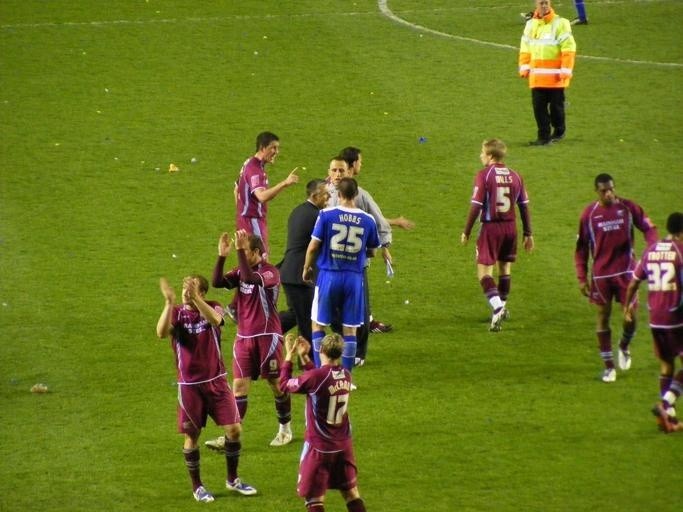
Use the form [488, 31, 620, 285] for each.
[602, 367, 617, 384]
[527, 138, 553, 146]
[225, 476, 258, 496]
[269, 432, 293, 446]
[370, 318, 393, 334]
[489, 306, 509, 333]
[192, 485, 215, 503]
[550, 132, 567, 141]
[205, 435, 226, 453]
[651, 401, 683, 435]
[618, 348, 631, 371]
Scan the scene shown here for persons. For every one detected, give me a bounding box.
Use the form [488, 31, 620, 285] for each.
[624, 213, 683, 433]
[519, 0, 576, 146]
[570, 0, 588, 26]
[575, 173, 659, 384]
[461, 136, 534, 332]
